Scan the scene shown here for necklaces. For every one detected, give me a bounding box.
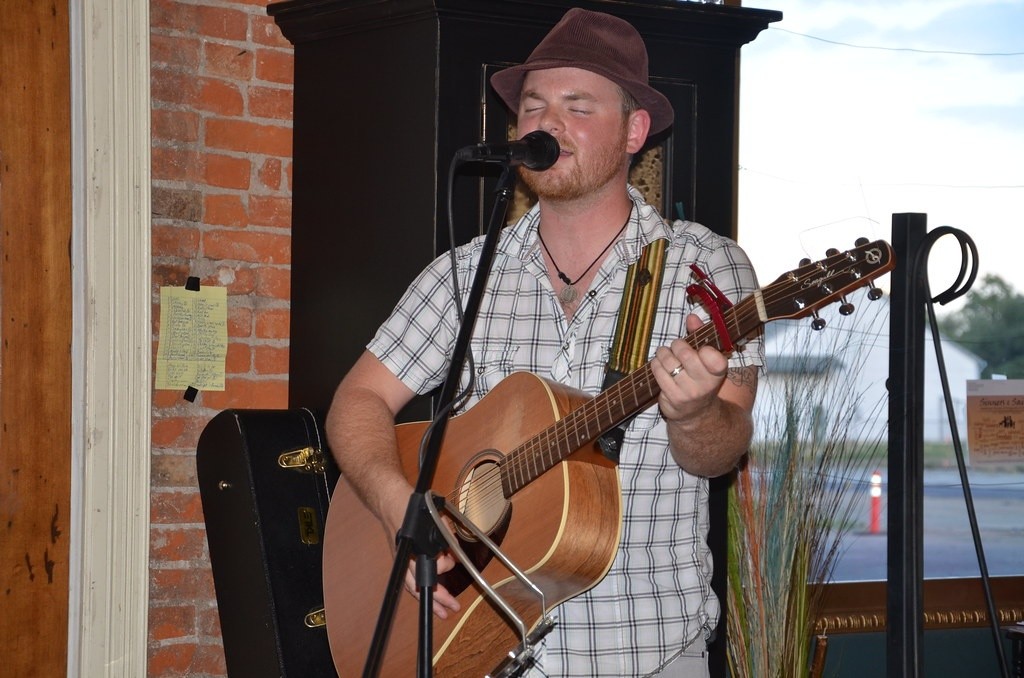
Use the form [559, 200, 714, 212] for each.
[535, 201, 634, 312]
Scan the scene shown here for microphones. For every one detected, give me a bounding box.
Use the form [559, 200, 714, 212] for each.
[456, 130, 561, 170]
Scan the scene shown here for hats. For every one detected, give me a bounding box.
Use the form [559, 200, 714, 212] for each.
[491, 8, 675, 138]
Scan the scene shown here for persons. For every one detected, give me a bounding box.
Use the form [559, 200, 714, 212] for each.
[325, 9, 767, 678]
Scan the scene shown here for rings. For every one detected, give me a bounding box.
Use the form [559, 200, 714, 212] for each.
[669, 363, 684, 379]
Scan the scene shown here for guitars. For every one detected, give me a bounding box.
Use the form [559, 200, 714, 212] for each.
[321, 235, 891, 678]
